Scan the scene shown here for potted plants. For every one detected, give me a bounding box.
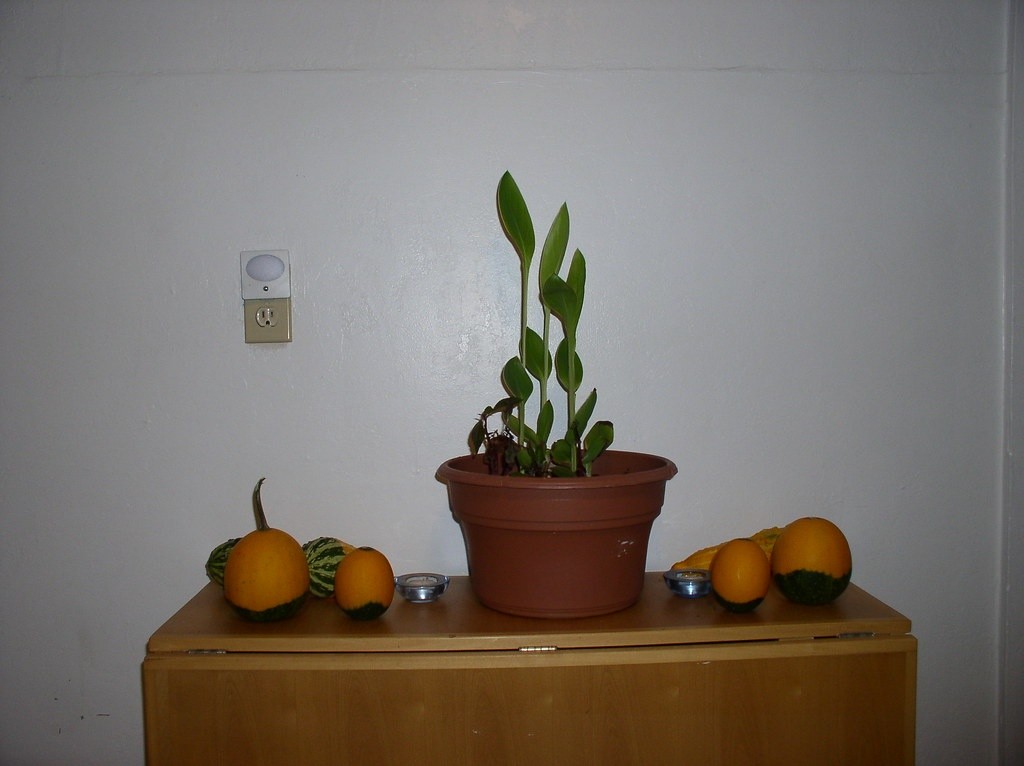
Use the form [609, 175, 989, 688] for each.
[435, 169, 678, 620]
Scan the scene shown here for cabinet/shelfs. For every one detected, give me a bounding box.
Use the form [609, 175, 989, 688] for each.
[140, 575, 918, 766]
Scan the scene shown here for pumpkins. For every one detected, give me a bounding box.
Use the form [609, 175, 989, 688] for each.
[670, 516, 852, 613]
[204, 477, 395, 620]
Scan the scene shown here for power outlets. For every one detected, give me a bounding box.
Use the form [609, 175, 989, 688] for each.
[244, 297, 294, 343]
